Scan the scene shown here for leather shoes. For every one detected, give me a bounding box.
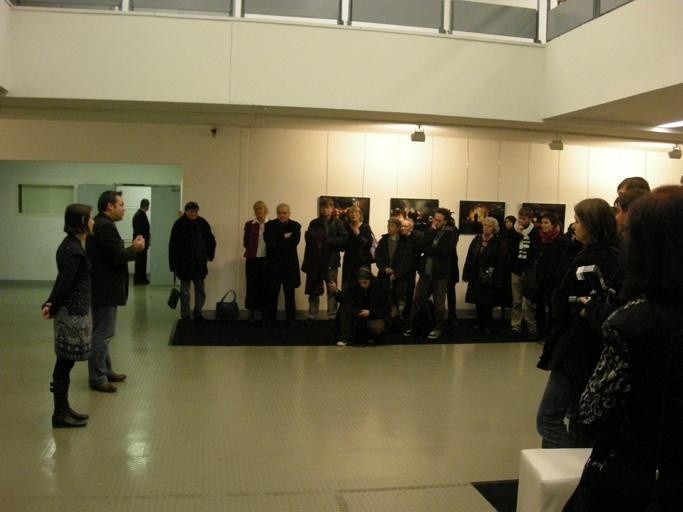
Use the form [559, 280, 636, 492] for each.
[108, 373, 127, 382]
[92, 380, 118, 393]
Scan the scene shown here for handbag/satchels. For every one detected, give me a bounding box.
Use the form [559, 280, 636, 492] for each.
[167, 288, 180, 308]
[368, 237, 377, 263]
[215, 301, 238, 318]
[479, 266, 496, 281]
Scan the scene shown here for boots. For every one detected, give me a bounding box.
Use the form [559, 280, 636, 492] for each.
[49, 372, 87, 428]
[65, 378, 89, 421]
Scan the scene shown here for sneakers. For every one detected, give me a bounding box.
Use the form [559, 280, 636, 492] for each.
[512, 327, 521, 333]
[327, 312, 335, 318]
[403, 329, 415, 336]
[427, 330, 440, 338]
[307, 313, 318, 319]
[338, 338, 353, 347]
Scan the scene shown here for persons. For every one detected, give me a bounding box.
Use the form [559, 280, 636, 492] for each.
[400, 218, 414, 238]
[340, 206, 376, 295]
[263, 203, 301, 325]
[374, 217, 415, 332]
[461, 217, 507, 333]
[445, 215, 459, 327]
[243, 201, 269, 322]
[403, 208, 459, 339]
[499, 216, 523, 321]
[617, 177, 651, 198]
[301, 197, 350, 322]
[537, 198, 623, 449]
[614, 188, 649, 241]
[328, 266, 391, 346]
[41, 204, 95, 429]
[536, 211, 575, 345]
[132, 199, 150, 285]
[561, 186, 682, 512]
[85, 191, 146, 393]
[168, 202, 216, 320]
[505, 207, 539, 338]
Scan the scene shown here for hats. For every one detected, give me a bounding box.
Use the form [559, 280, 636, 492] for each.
[357, 266, 370, 280]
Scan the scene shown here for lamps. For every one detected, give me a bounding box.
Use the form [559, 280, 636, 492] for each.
[549, 141, 563, 150]
[668, 150, 681, 159]
[411, 132, 425, 142]
